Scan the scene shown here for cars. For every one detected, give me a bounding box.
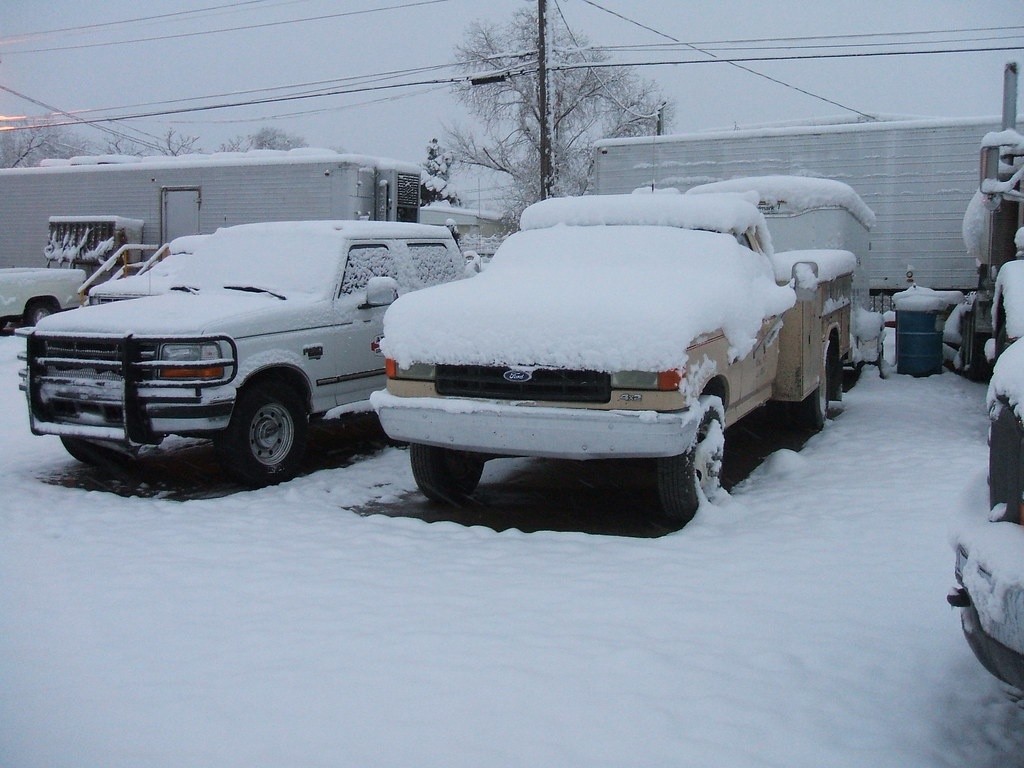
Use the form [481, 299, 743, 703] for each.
[88, 254, 193, 306]
[953, 227, 1024, 686]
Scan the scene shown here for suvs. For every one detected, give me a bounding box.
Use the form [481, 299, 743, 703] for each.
[12, 218, 483, 488]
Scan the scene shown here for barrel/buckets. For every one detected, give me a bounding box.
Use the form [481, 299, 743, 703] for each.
[895, 311, 945, 377]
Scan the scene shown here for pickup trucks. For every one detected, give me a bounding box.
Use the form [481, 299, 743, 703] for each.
[380, 195, 854, 522]
[0, 268, 86, 330]
